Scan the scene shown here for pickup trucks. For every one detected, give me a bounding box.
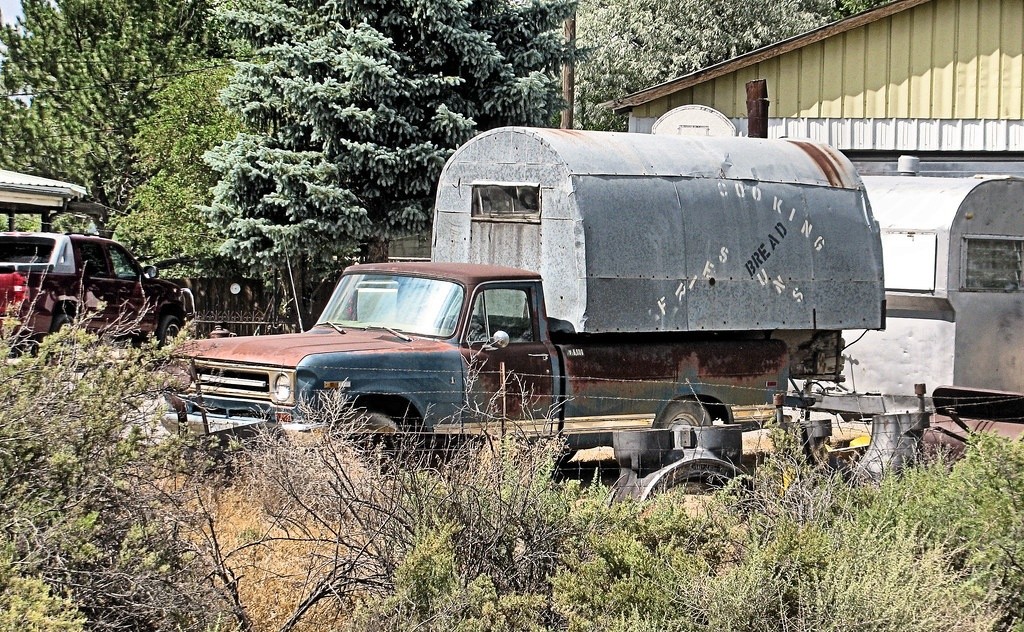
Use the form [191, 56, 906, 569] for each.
[0, 232, 188, 351]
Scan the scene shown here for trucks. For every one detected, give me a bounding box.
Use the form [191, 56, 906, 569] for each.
[150, 126, 889, 521]
